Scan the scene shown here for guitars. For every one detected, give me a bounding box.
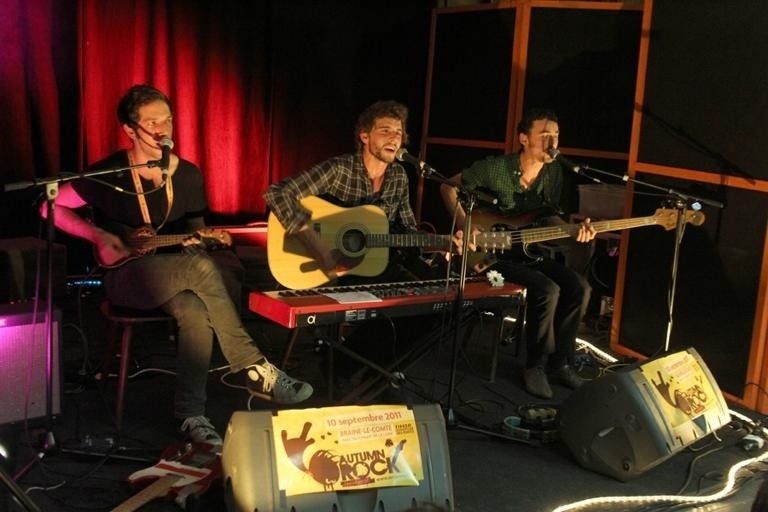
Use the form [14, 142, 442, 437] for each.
[107, 439, 223, 512]
[267, 195, 513, 290]
[457, 196, 705, 274]
[94, 219, 233, 269]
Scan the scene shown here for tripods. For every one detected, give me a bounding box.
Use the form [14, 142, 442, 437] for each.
[0, 168, 154, 489]
[437, 185, 545, 453]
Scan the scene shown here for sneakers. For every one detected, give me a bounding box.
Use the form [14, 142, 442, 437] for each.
[177, 411, 224, 453]
[243, 356, 314, 404]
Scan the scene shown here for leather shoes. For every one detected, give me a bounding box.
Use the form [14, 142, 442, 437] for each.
[522, 364, 585, 399]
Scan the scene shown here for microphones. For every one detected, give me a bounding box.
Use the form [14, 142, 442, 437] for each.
[156, 137, 176, 185]
[547, 146, 584, 179]
[392, 145, 434, 177]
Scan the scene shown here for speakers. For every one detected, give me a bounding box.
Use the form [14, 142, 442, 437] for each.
[1, 297, 67, 429]
[555, 344, 732, 482]
[221, 402, 459, 511]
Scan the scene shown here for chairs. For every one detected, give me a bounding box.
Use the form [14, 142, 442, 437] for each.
[87, 206, 525, 435]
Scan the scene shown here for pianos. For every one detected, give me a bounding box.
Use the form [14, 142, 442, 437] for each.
[247, 275, 528, 330]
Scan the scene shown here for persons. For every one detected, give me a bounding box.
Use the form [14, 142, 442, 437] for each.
[38, 86, 316, 457]
[255, 98, 478, 406]
[439, 112, 598, 401]
